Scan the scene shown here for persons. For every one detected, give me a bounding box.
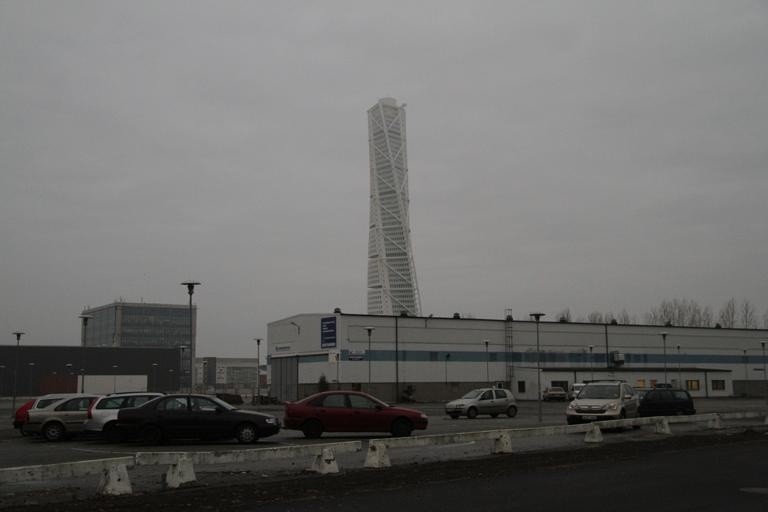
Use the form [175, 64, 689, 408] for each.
[317, 375, 328, 393]
[400, 384, 416, 403]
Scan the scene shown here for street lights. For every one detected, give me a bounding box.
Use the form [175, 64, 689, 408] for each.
[180, 282, 201, 390]
[252, 338, 264, 395]
[363, 327, 376, 390]
[12, 331, 26, 396]
[529, 313, 546, 422]
[79, 315, 93, 391]
[659, 331, 669, 383]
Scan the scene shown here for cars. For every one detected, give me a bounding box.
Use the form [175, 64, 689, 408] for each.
[543, 381, 696, 425]
[445, 388, 517, 419]
